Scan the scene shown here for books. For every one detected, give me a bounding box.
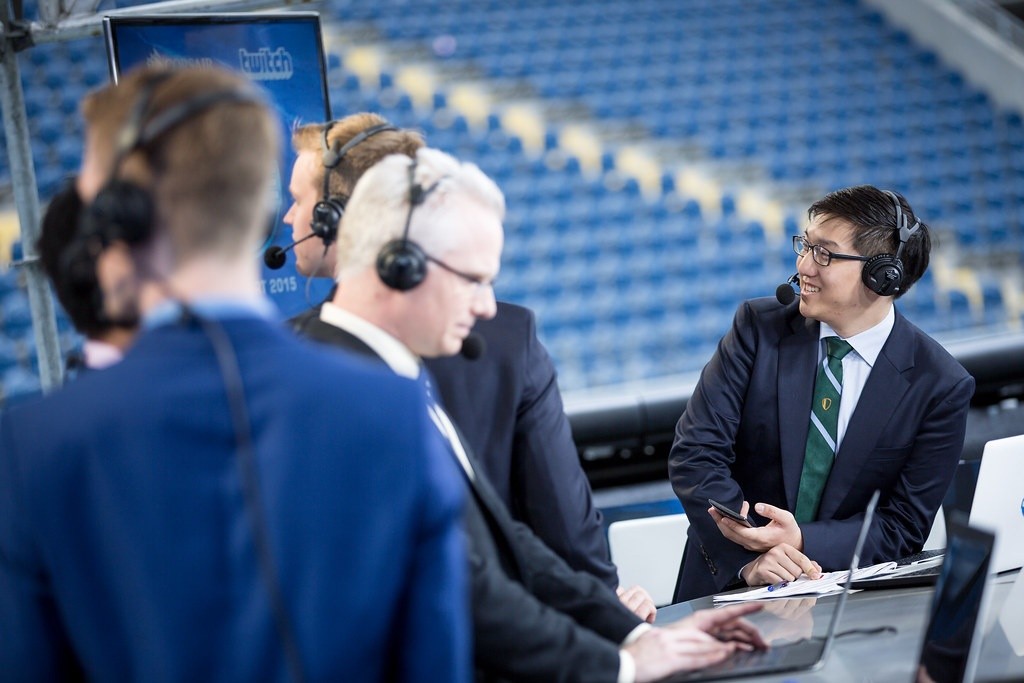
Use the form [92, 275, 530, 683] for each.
[712, 561, 898, 602]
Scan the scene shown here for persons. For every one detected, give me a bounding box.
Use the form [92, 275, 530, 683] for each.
[301, 145, 770, 683]
[665, 183, 976, 604]
[0, 59, 475, 683]
[32, 187, 141, 374]
[275, 113, 658, 625]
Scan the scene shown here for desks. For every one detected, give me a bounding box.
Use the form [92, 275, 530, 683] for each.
[640, 546, 1024, 683]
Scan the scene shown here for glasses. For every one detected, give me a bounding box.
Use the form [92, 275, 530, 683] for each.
[792, 234, 873, 267]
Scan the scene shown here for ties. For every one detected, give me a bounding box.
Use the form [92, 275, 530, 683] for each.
[794, 337, 854, 525]
[417, 364, 435, 409]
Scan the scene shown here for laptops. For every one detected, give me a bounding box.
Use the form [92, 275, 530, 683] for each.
[652, 489, 880, 683]
[837, 434, 1024, 590]
[908, 510, 1000, 683]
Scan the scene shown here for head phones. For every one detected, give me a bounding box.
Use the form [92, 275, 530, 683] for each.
[311, 120, 400, 246]
[84, 65, 259, 247]
[377, 158, 451, 291]
[862, 190, 921, 296]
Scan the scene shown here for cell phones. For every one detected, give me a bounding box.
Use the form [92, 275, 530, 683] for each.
[709, 499, 753, 528]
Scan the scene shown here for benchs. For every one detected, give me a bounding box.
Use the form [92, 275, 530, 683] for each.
[0, 0, 1023, 421]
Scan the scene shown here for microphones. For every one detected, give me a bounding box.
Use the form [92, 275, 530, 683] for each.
[775, 273, 799, 305]
[265, 224, 328, 270]
